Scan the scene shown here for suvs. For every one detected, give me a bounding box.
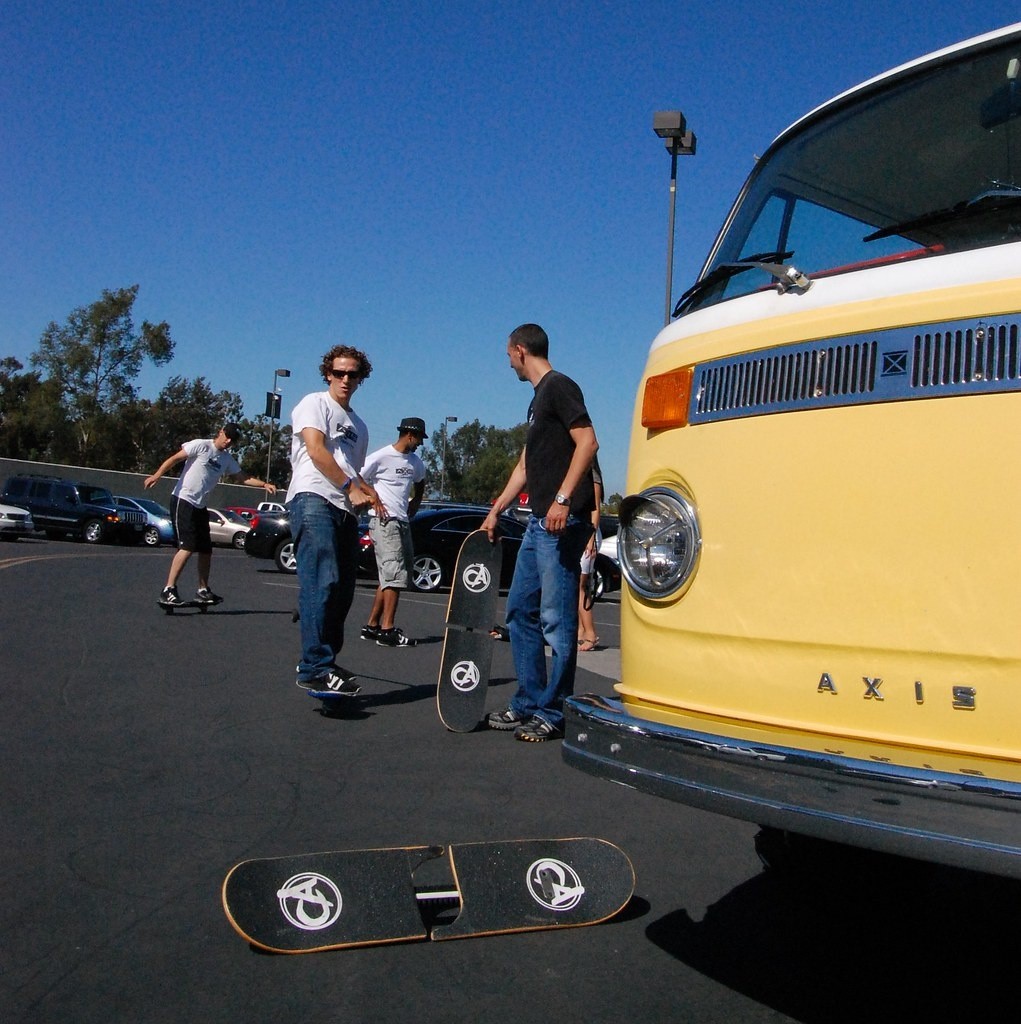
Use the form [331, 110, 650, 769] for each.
[0, 473, 148, 544]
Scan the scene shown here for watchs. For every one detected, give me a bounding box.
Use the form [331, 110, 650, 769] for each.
[554, 494, 571, 507]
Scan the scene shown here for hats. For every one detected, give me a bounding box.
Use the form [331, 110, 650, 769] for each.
[223, 423, 241, 443]
[397, 418, 428, 439]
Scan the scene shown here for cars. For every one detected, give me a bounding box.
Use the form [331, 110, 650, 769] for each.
[207, 508, 253, 550]
[598, 515, 619, 567]
[0, 504, 34, 541]
[245, 510, 371, 574]
[227, 508, 259, 528]
[357, 508, 616, 595]
[91, 495, 174, 547]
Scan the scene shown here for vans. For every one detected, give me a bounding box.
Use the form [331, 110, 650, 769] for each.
[563, 21, 1021, 877]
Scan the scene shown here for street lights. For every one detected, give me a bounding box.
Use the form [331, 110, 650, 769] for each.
[653, 112, 697, 327]
[265, 369, 290, 502]
[440, 417, 457, 501]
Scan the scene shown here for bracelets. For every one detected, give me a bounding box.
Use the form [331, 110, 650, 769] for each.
[263, 483, 270, 488]
[341, 477, 352, 490]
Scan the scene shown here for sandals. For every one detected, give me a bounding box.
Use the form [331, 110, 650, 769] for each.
[576, 636, 599, 651]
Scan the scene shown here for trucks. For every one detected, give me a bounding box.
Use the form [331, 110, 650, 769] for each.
[256, 502, 289, 512]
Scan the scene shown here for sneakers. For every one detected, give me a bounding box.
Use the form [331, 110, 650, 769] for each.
[484, 706, 523, 730]
[513, 715, 565, 743]
[376, 628, 417, 647]
[192, 587, 223, 603]
[296, 660, 356, 681]
[360, 625, 382, 641]
[159, 584, 185, 606]
[296, 671, 362, 694]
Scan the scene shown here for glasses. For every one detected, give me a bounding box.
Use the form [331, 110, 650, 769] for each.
[330, 370, 361, 380]
[223, 429, 234, 441]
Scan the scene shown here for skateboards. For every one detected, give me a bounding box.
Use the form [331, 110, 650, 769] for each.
[308, 691, 346, 714]
[436, 528, 503, 733]
[221, 837, 636, 955]
[157, 600, 224, 614]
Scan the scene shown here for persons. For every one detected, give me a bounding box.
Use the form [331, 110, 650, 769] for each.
[284, 345, 375, 695]
[480, 323, 600, 743]
[359, 417, 429, 647]
[143, 422, 276, 605]
[576, 453, 604, 651]
